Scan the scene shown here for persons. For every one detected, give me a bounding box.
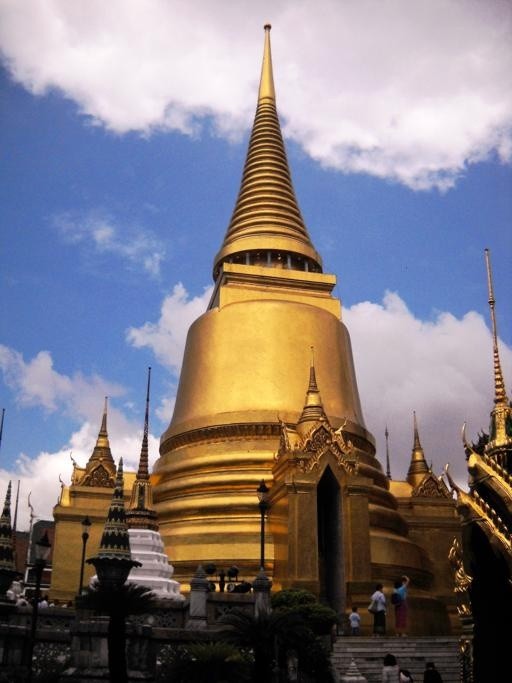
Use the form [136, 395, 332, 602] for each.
[16, 594, 73, 608]
[399, 669, 414, 683]
[382, 654, 401, 683]
[370, 584, 387, 635]
[393, 575, 410, 638]
[349, 606, 362, 635]
[423, 662, 443, 683]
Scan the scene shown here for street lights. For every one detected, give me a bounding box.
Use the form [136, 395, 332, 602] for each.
[24, 529, 54, 676]
[255, 477, 270, 579]
[76, 517, 91, 597]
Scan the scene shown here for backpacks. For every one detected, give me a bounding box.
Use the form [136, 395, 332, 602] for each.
[391, 593, 401, 604]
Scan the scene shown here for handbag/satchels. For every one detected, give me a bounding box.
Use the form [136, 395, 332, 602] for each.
[368, 600, 377, 614]
